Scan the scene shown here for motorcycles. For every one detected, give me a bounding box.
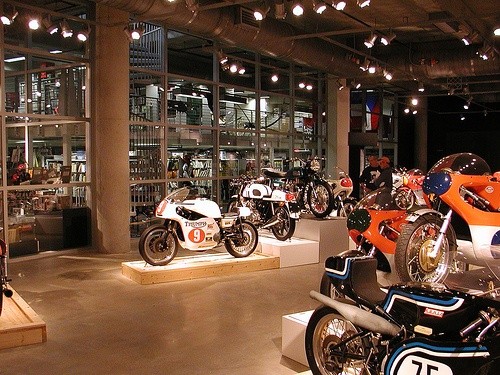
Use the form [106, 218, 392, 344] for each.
[303, 249, 500, 375]
[326, 152, 500, 285]
[139, 186, 259, 267]
[260, 155, 334, 217]
[228, 166, 301, 242]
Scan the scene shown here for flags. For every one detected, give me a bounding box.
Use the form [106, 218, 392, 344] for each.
[365, 93, 381, 130]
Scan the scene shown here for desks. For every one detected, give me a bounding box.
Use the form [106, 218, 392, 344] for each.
[34, 207, 91, 251]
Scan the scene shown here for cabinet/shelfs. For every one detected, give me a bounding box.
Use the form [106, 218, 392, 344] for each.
[43, 148, 288, 217]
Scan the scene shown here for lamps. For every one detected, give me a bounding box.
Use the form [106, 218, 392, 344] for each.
[461, 27, 500, 60]
[1, 5, 92, 42]
[253, 0, 370, 21]
[214, 49, 313, 91]
[336, 58, 424, 115]
[364, 27, 379, 48]
[130, 25, 145, 40]
[380, 25, 396, 45]
[463, 98, 472, 109]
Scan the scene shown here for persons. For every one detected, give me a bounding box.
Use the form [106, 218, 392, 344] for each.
[358, 155, 382, 194]
[372, 156, 393, 188]
[179, 156, 195, 178]
